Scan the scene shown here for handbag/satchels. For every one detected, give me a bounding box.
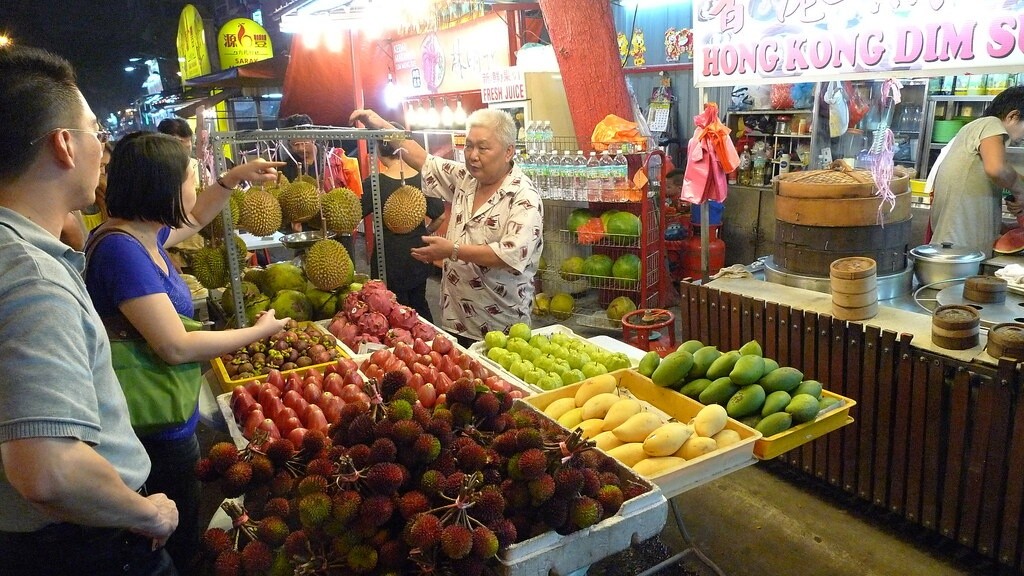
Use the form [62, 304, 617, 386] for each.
[80, 231, 205, 427]
[844, 81, 869, 128]
[824, 81, 849, 138]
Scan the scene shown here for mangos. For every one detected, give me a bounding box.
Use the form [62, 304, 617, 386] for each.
[545, 374, 742, 478]
[637, 340, 838, 438]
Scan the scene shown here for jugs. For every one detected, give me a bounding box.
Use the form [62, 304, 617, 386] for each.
[843, 127, 868, 159]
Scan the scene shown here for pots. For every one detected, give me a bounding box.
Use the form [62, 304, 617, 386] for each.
[910, 242, 985, 290]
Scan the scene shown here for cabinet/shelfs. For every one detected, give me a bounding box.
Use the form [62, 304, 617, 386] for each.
[722, 109, 814, 265]
[516, 150, 667, 343]
[922, 94, 1024, 183]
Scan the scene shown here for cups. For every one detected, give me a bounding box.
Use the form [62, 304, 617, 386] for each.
[776, 122, 790, 135]
[910, 139, 918, 161]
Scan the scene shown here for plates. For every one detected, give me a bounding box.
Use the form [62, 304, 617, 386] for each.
[932, 116, 975, 143]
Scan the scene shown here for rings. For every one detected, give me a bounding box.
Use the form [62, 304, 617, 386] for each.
[426, 261, 429, 263]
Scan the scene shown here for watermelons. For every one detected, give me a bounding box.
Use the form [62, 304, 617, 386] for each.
[991, 226, 1024, 253]
[536, 209, 643, 287]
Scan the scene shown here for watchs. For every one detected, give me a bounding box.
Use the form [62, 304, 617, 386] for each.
[215, 172, 235, 192]
[449, 240, 460, 262]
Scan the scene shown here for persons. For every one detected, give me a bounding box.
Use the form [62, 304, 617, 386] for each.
[348, 107, 546, 351]
[359, 120, 446, 324]
[81, 132, 291, 576]
[0, 39, 179, 576]
[926, 82, 1024, 276]
[277, 113, 355, 270]
[158, 120, 213, 187]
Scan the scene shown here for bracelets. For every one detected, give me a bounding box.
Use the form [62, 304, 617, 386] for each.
[426, 224, 432, 234]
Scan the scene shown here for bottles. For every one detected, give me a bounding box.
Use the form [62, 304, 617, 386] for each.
[728, 145, 765, 187]
[513, 120, 628, 203]
[898, 104, 921, 131]
[778, 154, 790, 175]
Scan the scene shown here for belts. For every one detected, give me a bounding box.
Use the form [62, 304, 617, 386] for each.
[0, 488, 147, 545]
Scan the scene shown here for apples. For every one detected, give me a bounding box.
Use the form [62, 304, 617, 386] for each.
[485, 322, 632, 391]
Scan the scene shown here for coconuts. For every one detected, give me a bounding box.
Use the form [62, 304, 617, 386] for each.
[532, 292, 637, 328]
[219, 262, 368, 327]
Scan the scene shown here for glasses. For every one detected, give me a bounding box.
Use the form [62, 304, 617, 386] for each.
[30, 128, 112, 144]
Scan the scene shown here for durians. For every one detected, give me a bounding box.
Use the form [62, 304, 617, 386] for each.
[190, 170, 427, 292]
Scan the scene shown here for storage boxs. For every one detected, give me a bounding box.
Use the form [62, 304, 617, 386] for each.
[207, 329, 857, 574]
